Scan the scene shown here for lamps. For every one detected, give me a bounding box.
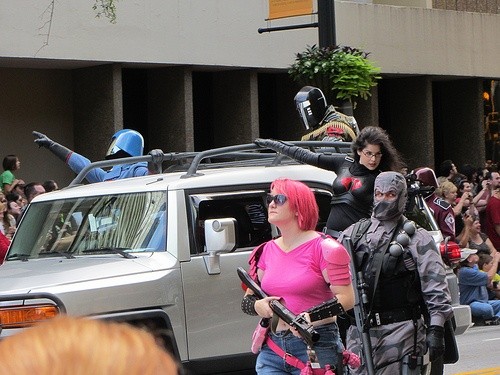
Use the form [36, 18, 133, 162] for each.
[480, 91, 490, 104]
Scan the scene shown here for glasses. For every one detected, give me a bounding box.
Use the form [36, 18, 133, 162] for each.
[265, 193, 288, 205]
[358, 149, 383, 158]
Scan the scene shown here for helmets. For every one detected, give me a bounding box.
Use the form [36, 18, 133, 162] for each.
[294, 86, 328, 131]
[105, 129, 144, 155]
[373, 171, 408, 220]
[410, 168, 439, 189]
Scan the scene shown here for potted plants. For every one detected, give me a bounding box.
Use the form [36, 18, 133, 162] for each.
[287, 43, 383, 101]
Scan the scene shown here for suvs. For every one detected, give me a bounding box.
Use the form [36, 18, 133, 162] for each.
[0, 141, 471, 375]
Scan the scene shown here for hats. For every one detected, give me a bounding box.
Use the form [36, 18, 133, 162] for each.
[459, 248, 478, 263]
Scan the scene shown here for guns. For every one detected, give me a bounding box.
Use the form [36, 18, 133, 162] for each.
[342, 236, 378, 375]
[237, 266, 320, 348]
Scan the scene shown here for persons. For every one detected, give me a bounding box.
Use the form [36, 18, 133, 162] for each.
[1, 129, 176, 375]
[242, 86, 500, 375]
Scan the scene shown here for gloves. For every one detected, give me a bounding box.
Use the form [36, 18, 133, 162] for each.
[254, 137, 321, 168]
[31, 130, 70, 162]
[423, 326, 445, 362]
[148, 149, 165, 173]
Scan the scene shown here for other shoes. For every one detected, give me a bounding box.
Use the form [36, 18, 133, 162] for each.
[484, 319, 499, 325]
